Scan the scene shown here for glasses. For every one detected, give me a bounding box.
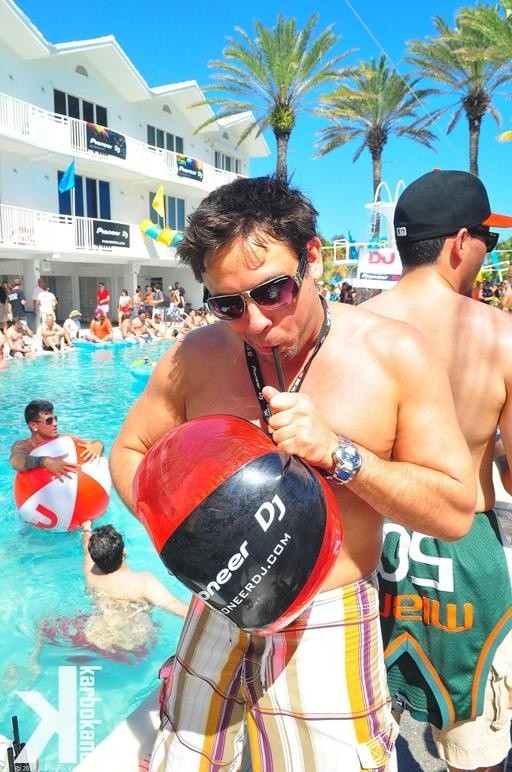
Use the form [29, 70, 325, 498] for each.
[470, 230, 501, 254]
[39, 416, 57, 425]
[202, 254, 309, 321]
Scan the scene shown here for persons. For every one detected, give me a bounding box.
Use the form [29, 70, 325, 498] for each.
[352, 169, 512, 772]
[469, 276, 512, 312]
[7, 520, 192, 691]
[316, 278, 356, 303]
[1, 278, 220, 367]
[11, 398, 103, 483]
[107, 172, 476, 772]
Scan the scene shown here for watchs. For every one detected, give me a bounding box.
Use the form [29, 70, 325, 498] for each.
[326, 432, 362, 485]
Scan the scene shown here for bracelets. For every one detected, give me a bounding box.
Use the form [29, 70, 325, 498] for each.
[492, 433, 500, 442]
[82, 530, 92, 534]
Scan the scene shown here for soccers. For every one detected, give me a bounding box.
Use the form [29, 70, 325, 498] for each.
[16, 435, 109, 530]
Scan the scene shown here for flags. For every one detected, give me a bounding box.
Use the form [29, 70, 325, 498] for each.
[151, 185, 165, 217]
[59, 159, 74, 193]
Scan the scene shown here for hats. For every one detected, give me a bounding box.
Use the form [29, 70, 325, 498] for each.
[93, 308, 102, 315]
[395, 170, 512, 242]
[70, 309, 81, 318]
